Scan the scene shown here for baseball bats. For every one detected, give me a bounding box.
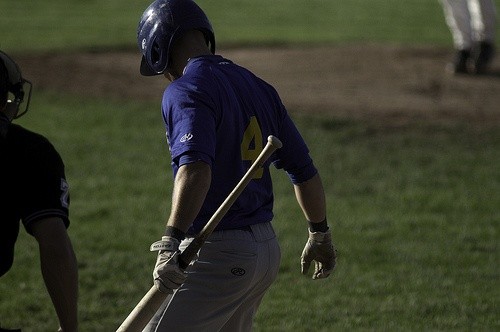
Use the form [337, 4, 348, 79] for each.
[117, 135, 283, 332]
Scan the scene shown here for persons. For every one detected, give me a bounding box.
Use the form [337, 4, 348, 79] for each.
[0, 51, 80, 332]
[438, 0, 494, 74]
[138, 0, 337, 332]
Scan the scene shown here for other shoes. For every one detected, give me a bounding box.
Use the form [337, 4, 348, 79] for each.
[455, 41, 492, 76]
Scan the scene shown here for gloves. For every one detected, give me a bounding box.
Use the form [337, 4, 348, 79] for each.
[300, 227, 336, 280]
[150, 236, 185, 294]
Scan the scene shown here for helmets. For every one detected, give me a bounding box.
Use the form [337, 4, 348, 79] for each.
[0, 50, 32, 121]
[136, 1, 216, 77]
